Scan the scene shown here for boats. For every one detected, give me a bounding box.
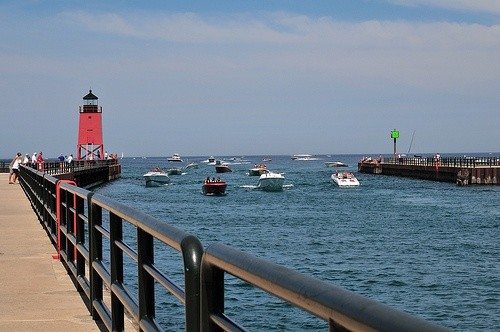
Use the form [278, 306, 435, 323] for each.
[324, 161, 346, 166]
[143, 170, 171, 186]
[222, 157, 252, 165]
[332, 173, 360, 187]
[258, 172, 285, 188]
[187, 162, 199, 169]
[249, 167, 269, 175]
[214, 160, 233, 172]
[263, 159, 272, 162]
[168, 168, 181, 175]
[209, 155, 215, 162]
[290, 154, 312, 159]
[202, 177, 227, 194]
[167, 153, 183, 162]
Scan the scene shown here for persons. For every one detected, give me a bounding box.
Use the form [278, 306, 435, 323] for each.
[24, 153, 30, 166]
[32, 152, 44, 169]
[9, 153, 24, 184]
[105, 151, 113, 159]
[58, 153, 73, 170]
[335, 170, 352, 178]
[155, 167, 165, 173]
[205, 176, 220, 183]
[255, 163, 266, 168]
[363, 154, 382, 163]
[92, 150, 100, 159]
[433, 153, 440, 165]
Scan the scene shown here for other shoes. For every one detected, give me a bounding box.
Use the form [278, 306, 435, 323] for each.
[9, 182, 14, 184]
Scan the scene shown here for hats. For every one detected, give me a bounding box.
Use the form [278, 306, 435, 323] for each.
[17, 152, 22, 157]
